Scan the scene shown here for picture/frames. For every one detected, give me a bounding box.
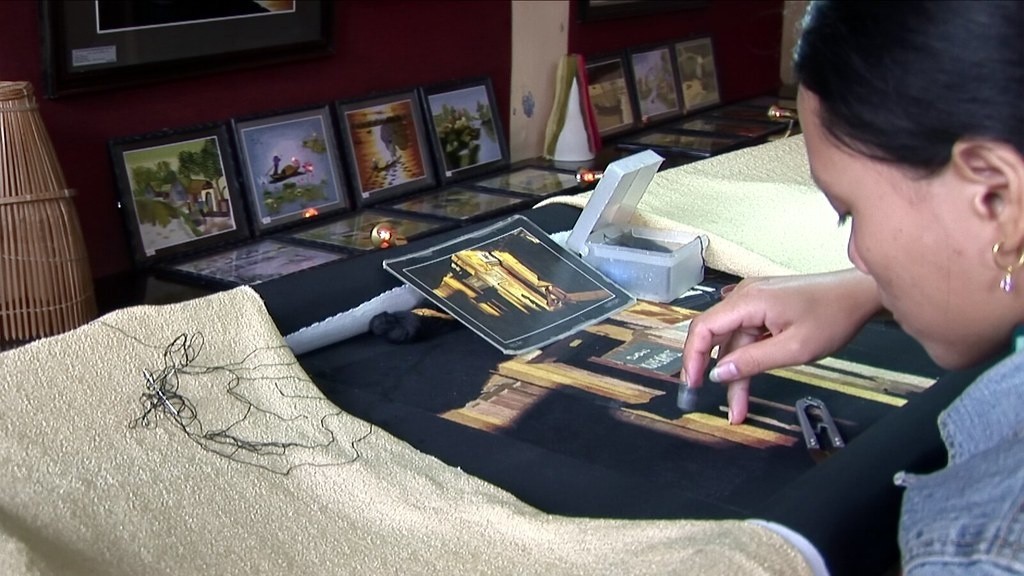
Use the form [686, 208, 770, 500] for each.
[673, 36, 722, 113]
[623, 39, 684, 128]
[54, 0, 327, 74]
[582, 52, 637, 139]
[576, 0, 708, 22]
[229, 99, 350, 237]
[419, 74, 509, 182]
[107, 122, 249, 266]
[330, 85, 441, 211]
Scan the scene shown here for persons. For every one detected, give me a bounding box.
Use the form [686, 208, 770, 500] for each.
[679, 0, 1024, 576]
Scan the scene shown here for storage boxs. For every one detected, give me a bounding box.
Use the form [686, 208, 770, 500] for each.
[570, 149, 705, 306]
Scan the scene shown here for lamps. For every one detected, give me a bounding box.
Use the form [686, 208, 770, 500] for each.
[0, 80, 98, 340]
